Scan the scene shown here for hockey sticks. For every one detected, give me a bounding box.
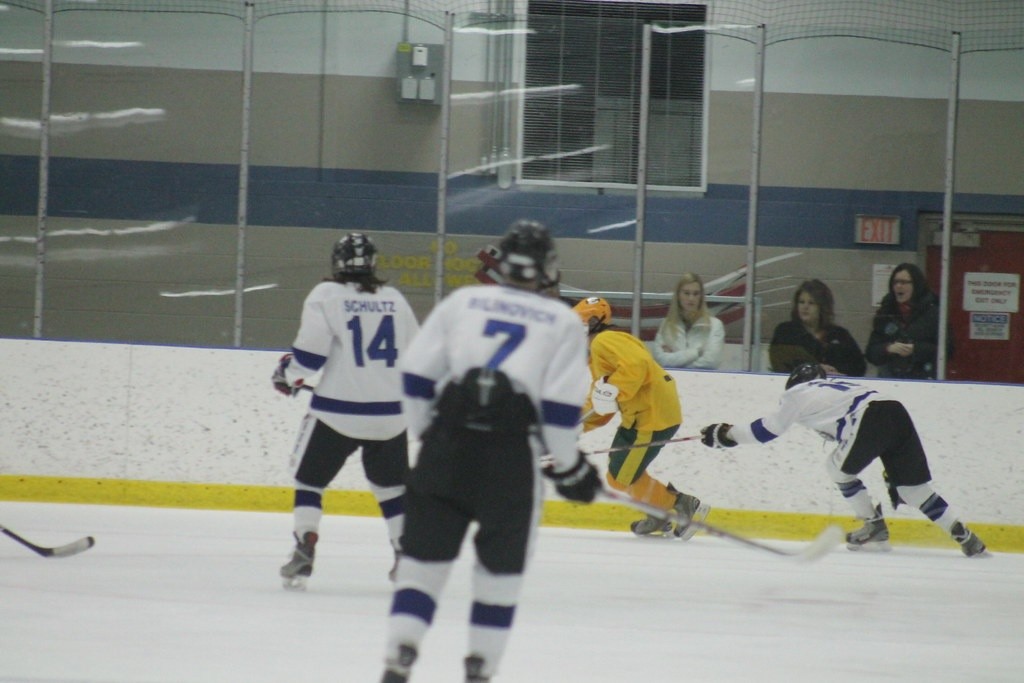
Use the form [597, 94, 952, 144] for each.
[299, 383, 314, 392]
[0, 523, 96, 560]
[596, 486, 846, 569]
[574, 409, 595, 426]
[536, 433, 706, 463]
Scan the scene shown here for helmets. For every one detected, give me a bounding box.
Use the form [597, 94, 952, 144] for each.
[331, 233, 378, 278]
[785, 363, 826, 391]
[572, 295, 612, 329]
[484, 218, 561, 291]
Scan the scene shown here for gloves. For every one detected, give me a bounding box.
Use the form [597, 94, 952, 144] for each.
[700, 422, 738, 448]
[545, 452, 602, 502]
[884, 469, 905, 509]
[271, 354, 303, 397]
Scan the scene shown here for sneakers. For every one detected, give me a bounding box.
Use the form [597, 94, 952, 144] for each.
[844, 503, 890, 551]
[665, 481, 711, 542]
[279, 530, 320, 592]
[962, 531, 990, 558]
[388, 538, 403, 580]
[630, 512, 672, 537]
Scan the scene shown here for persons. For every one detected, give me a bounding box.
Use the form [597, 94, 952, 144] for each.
[381, 220, 600, 683]
[272, 232, 418, 593]
[866, 263, 955, 379]
[654, 274, 726, 370]
[769, 279, 867, 378]
[701, 364, 989, 558]
[571, 298, 710, 539]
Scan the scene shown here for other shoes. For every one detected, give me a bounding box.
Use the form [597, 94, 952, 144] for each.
[381, 645, 416, 683]
[462, 655, 491, 681]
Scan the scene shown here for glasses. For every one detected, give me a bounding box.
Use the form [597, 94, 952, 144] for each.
[891, 279, 914, 285]
